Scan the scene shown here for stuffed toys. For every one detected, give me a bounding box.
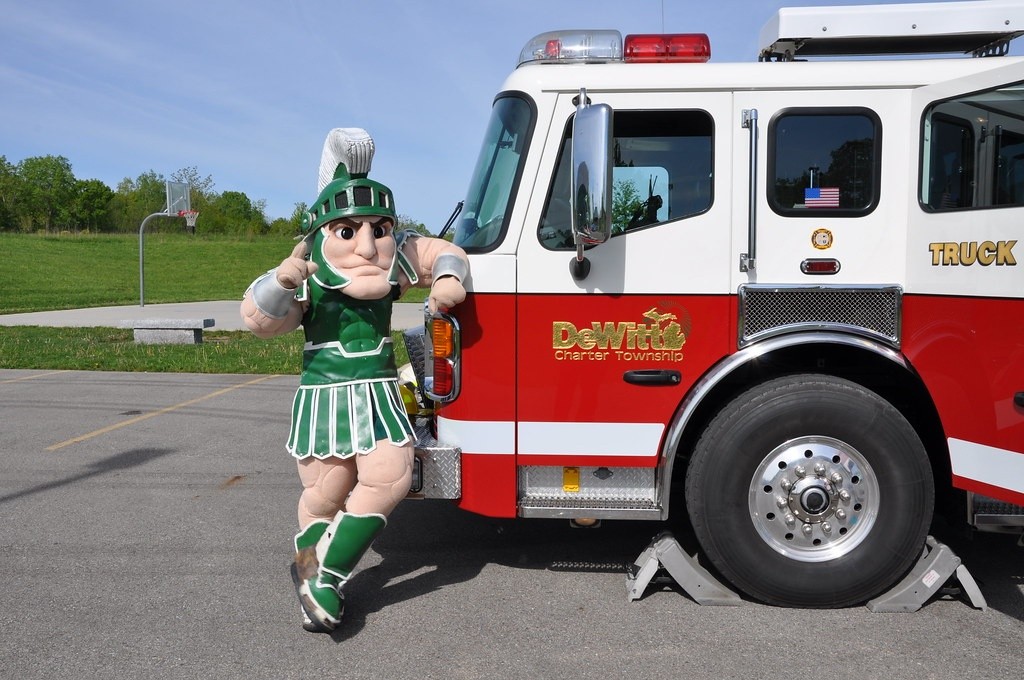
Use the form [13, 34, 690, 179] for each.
[239, 128, 470, 637]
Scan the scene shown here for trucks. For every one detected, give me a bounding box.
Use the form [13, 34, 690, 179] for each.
[398, 1, 1024, 609]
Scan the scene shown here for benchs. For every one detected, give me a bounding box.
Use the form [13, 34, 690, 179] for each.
[120, 318, 216, 344]
